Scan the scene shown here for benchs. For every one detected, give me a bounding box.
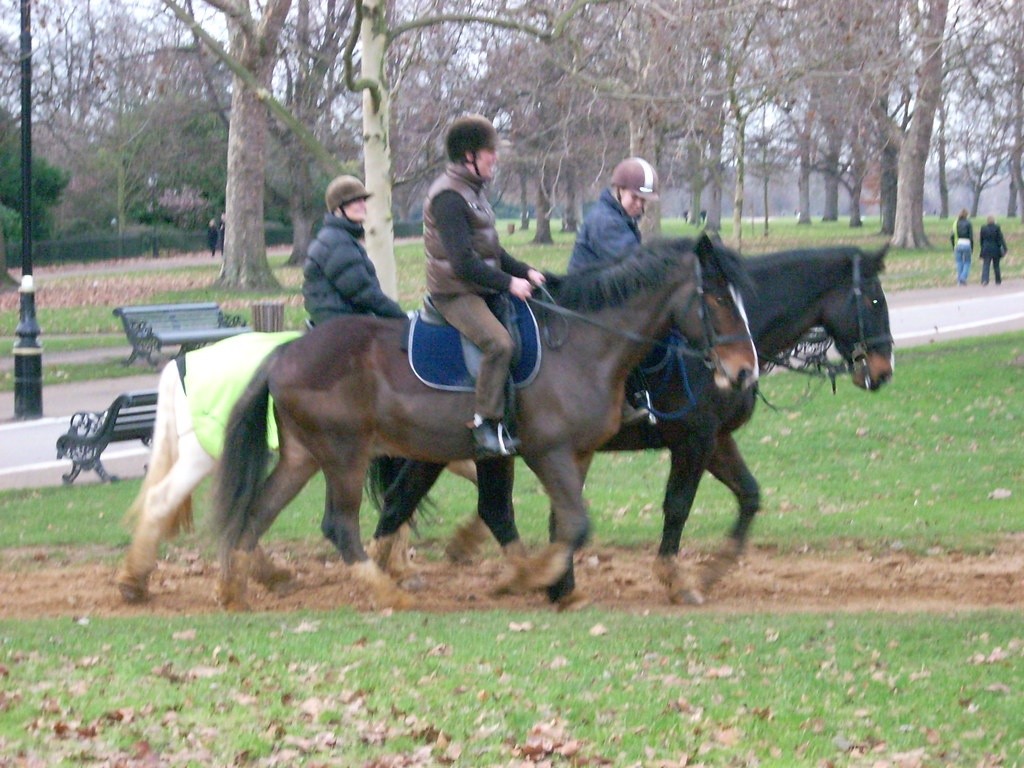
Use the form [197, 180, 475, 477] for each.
[113, 302, 251, 367]
[55, 390, 157, 484]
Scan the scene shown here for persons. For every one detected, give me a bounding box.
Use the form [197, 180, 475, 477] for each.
[682, 208, 708, 223]
[980, 215, 1007, 287]
[950, 206, 973, 287]
[217, 210, 225, 255]
[423, 112, 549, 451]
[566, 157, 662, 420]
[208, 219, 219, 256]
[304, 178, 410, 326]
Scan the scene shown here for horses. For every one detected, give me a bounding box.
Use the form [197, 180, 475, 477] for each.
[116, 229, 895, 616]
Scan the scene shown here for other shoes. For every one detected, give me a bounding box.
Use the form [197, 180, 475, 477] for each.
[619, 401, 649, 426]
[465, 418, 520, 460]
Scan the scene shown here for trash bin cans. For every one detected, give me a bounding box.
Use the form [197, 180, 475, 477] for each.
[251, 302, 285, 333]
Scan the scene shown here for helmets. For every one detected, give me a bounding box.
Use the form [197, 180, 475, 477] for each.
[325, 175, 371, 211]
[612, 156, 659, 201]
[445, 114, 499, 161]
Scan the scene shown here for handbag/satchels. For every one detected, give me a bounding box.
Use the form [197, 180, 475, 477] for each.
[998, 244, 1005, 257]
[955, 236, 971, 252]
